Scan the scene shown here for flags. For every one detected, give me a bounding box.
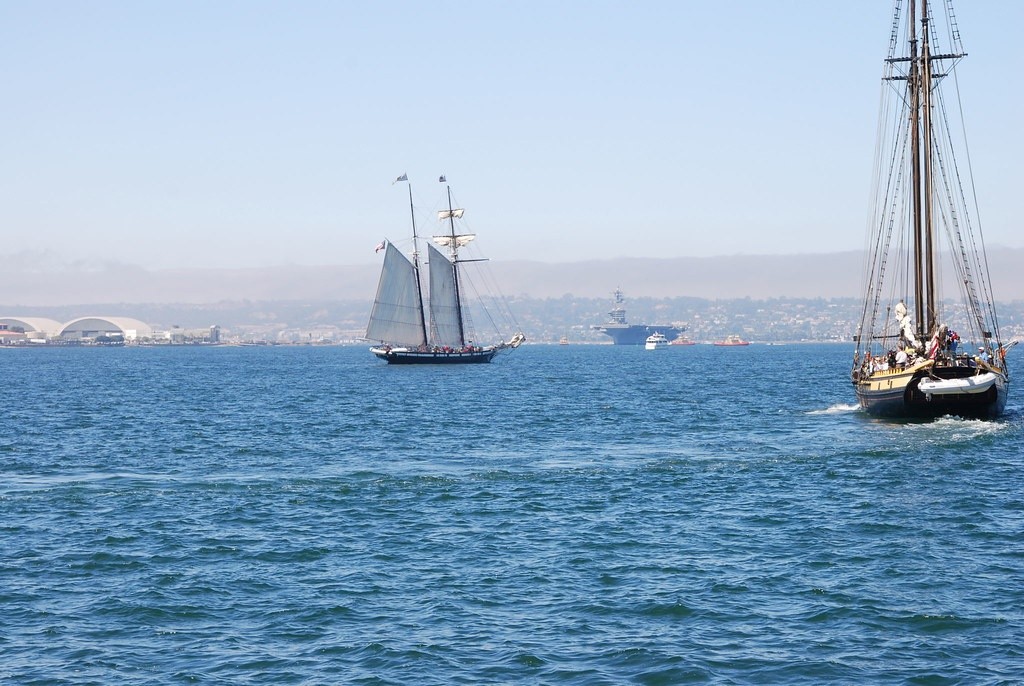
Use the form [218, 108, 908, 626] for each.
[438, 172, 447, 183]
[392, 172, 409, 184]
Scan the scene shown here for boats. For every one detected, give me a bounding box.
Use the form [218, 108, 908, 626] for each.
[645, 331, 668, 350]
[594, 286, 689, 344]
[714, 332, 750, 346]
[673, 333, 696, 345]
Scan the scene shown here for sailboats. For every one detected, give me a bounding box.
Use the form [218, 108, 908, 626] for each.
[850, 0, 1019, 420]
[366, 170, 526, 363]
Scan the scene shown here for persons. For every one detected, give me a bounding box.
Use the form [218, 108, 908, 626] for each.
[426, 344, 451, 353]
[949, 338, 957, 352]
[863, 345, 993, 377]
[464, 340, 474, 354]
